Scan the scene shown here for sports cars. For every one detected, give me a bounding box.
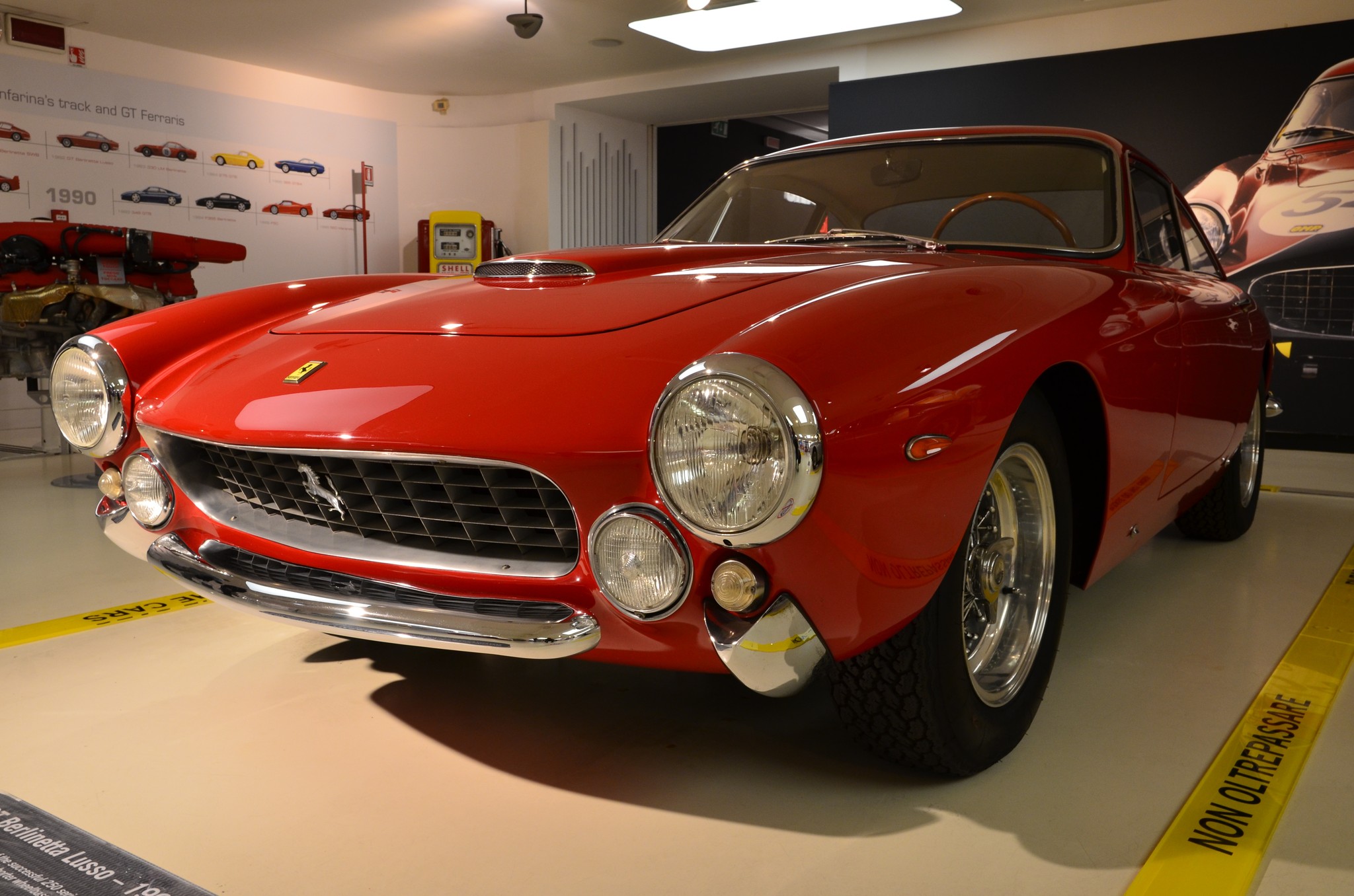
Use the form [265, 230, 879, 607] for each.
[0, 121, 30, 141]
[210, 150, 264, 170]
[262, 199, 314, 218]
[274, 157, 326, 176]
[0, 174, 20, 193]
[134, 141, 197, 162]
[1160, 55, 1354, 381]
[120, 185, 182, 206]
[46, 129, 1275, 786]
[196, 192, 251, 212]
[56, 129, 120, 152]
[322, 204, 371, 221]
